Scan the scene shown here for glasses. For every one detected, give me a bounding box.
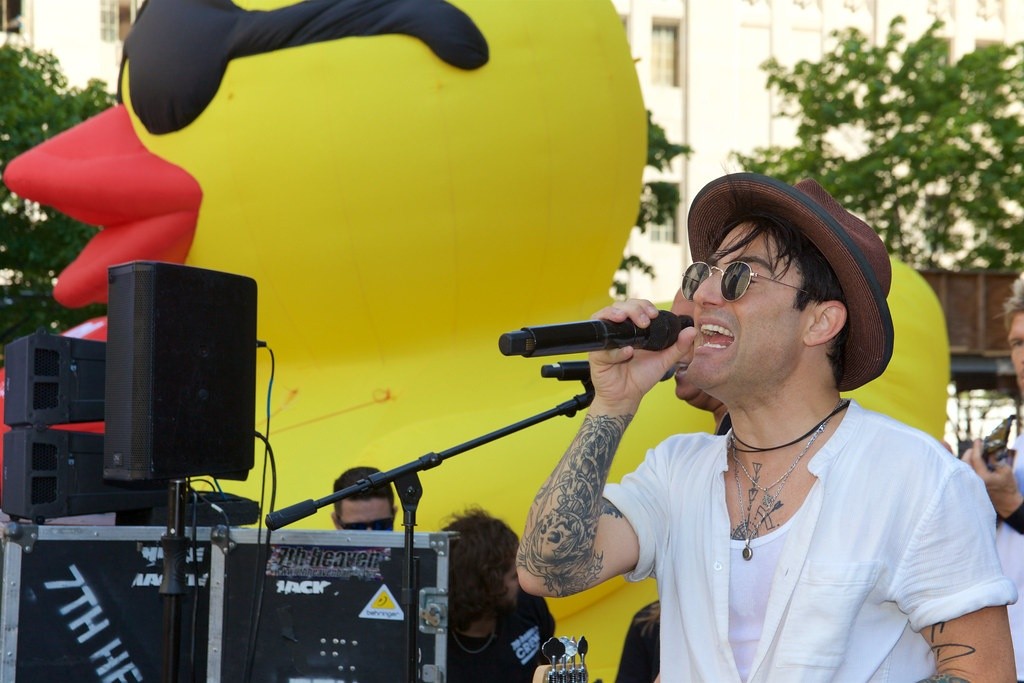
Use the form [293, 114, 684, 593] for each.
[680, 261, 809, 302]
[336, 517, 395, 531]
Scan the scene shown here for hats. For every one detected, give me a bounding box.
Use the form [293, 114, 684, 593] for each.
[688, 173, 895, 391]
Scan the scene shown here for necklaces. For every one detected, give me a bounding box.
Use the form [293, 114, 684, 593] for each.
[452, 619, 495, 654]
[729, 398, 851, 560]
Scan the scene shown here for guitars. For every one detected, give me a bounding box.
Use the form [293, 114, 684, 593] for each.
[983, 412, 1020, 469]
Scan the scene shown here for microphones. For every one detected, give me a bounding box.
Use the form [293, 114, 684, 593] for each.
[542, 360, 677, 381]
[498, 311, 680, 358]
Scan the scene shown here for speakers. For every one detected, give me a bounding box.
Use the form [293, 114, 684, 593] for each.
[0, 262, 258, 526]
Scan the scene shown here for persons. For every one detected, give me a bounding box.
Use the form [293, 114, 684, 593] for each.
[515, 172, 1017, 683]
[441, 505, 555, 683]
[331, 466, 397, 530]
[938, 268, 1024, 683]
